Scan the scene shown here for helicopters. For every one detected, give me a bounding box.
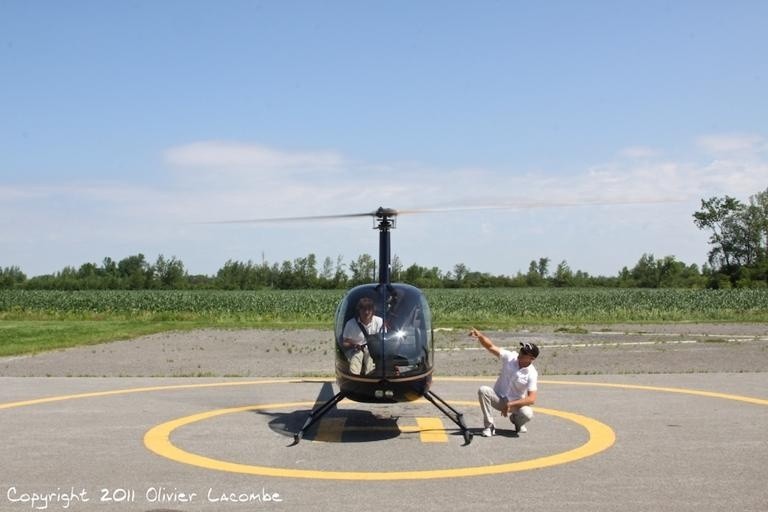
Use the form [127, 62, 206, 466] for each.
[191, 198, 690, 444]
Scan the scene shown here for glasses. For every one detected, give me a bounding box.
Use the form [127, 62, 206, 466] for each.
[520, 348, 529, 355]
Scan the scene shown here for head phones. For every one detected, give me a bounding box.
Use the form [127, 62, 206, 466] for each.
[355, 300, 377, 312]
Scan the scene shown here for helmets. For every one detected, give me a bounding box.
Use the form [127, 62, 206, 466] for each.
[355, 297, 376, 311]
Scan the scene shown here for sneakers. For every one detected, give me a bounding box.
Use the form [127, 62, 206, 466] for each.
[515, 424, 527, 433]
[482, 427, 495, 437]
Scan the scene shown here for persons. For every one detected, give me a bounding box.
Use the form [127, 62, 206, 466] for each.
[342, 297, 388, 376]
[470, 326, 539, 437]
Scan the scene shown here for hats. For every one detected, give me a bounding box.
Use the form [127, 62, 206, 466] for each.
[520, 342, 539, 358]
